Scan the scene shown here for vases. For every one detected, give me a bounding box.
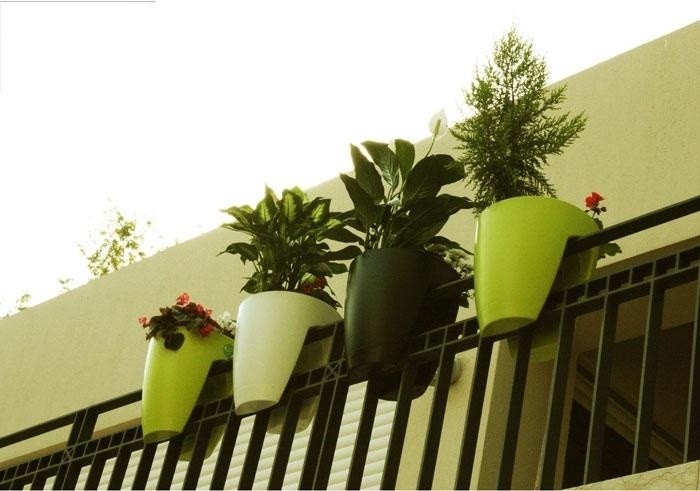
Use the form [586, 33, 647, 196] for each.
[343, 244, 461, 401]
[140, 326, 234, 463]
[232, 291, 344, 434]
[474, 195, 601, 364]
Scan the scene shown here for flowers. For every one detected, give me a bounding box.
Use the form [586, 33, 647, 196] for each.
[140, 291, 234, 352]
[330, 107, 475, 309]
[215, 182, 358, 310]
[445, 24, 623, 261]
